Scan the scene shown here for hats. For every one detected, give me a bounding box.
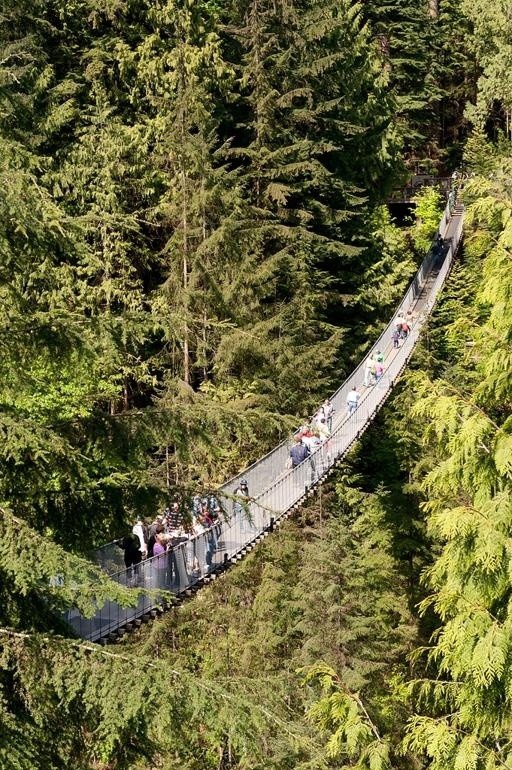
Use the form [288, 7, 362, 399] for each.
[240, 479, 247, 485]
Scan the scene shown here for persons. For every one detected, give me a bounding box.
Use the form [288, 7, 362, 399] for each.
[233, 480, 252, 533]
[289, 399, 335, 488]
[364, 350, 385, 389]
[435, 234, 445, 255]
[392, 310, 411, 348]
[346, 386, 360, 422]
[118, 491, 223, 587]
